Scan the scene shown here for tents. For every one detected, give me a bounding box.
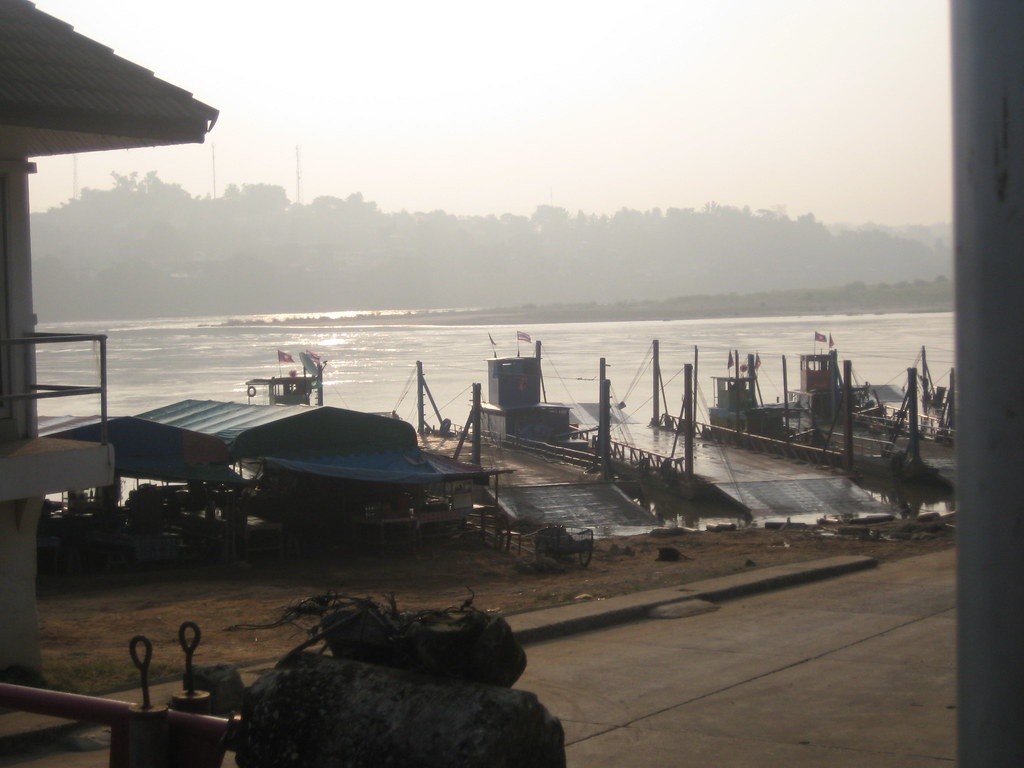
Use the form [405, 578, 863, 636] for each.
[36, 398, 517, 554]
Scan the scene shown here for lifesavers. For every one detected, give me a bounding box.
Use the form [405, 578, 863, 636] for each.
[246, 386, 257, 398]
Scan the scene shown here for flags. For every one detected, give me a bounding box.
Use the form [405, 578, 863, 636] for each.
[278, 350, 294, 362]
[815, 332, 827, 342]
[830, 334, 834, 347]
[728, 351, 734, 368]
[755, 354, 761, 369]
[517, 331, 531, 342]
[489, 334, 496, 345]
[306, 350, 320, 362]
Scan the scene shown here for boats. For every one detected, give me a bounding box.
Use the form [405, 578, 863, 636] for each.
[707, 350, 784, 439]
[786, 331, 887, 417]
[478, 330, 590, 449]
[245, 349, 328, 406]
